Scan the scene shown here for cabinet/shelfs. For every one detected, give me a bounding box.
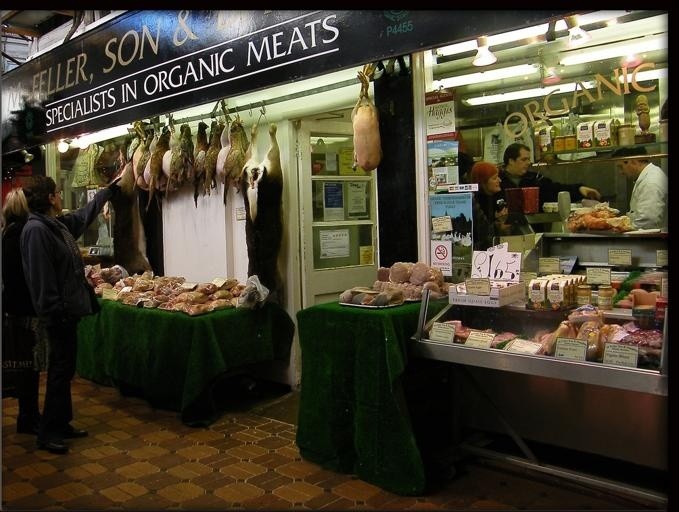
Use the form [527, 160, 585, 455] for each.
[423, 138, 668, 370]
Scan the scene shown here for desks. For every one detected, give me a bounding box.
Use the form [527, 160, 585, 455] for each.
[75, 298, 296, 427]
[297, 302, 470, 499]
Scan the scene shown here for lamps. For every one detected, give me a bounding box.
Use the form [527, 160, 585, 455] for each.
[472, 36, 498, 66]
[564, 13, 592, 48]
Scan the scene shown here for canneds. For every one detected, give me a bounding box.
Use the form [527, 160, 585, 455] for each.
[553, 136, 565, 151]
[565, 135, 577, 152]
[617, 125, 631, 146]
[598, 285, 613, 309]
[659, 119, 668, 144]
[576, 285, 591, 306]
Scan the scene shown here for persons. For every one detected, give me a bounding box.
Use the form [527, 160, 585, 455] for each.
[2, 186, 43, 436]
[21, 174, 122, 455]
[470, 161, 508, 223]
[611, 145, 668, 230]
[499, 143, 602, 213]
[458, 152, 494, 251]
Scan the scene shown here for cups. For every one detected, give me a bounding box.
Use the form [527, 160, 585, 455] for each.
[558, 191, 571, 221]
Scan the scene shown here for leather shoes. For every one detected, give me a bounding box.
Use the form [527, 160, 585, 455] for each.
[35, 435, 68, 454]
[17, 416, 42, 435]
[58, 419, 89, 439]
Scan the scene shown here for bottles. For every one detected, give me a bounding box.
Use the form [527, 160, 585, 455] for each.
[562, 276, 586, 305]
